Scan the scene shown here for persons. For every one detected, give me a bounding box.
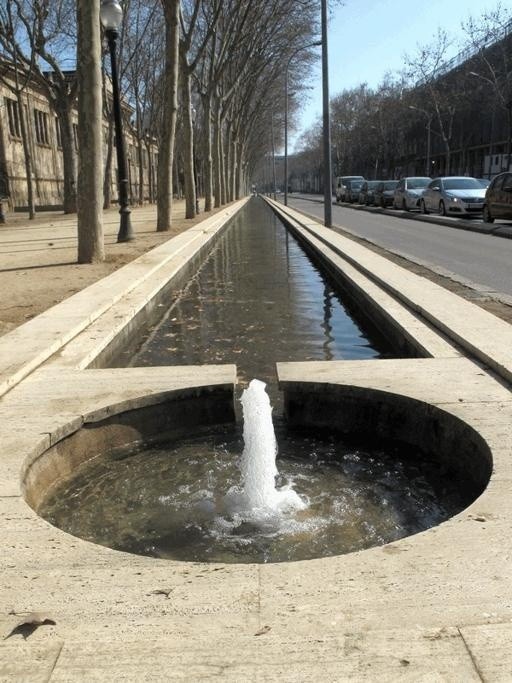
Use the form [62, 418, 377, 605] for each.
[340, 182, 347, 201]
[335, 183, 341, 203]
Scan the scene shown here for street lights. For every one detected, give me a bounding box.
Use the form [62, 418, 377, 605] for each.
[285, 40, 323, 207]
[371, 126, 380, 179]
[409, 106, 431, 177]
[98, 0, 136, 241]
[470, 71, 496, 154]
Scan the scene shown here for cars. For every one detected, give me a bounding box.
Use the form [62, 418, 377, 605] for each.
[337, 176, 434, 211]
[482, 171, 512, 223]
[420, 177, 489, 216]
